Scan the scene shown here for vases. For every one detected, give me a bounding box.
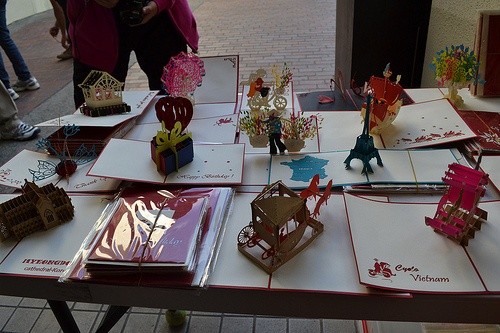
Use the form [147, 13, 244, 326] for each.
[285, 139, 305, 151]
[249, 135, 269, 147]
[442, 86, 464, 106]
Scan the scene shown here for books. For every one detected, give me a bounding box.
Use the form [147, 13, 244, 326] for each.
[469, 10, 500, 96]
[0, 54, 500, 299]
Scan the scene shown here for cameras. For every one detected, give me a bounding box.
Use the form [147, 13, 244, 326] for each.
[112, 0, 150, 25]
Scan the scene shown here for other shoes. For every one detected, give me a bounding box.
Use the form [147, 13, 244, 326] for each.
[56, 45, 73, 59]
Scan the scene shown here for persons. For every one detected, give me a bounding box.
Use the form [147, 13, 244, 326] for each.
[64, 0, 199, 111]
[49, 0, 70, 61]
[0, 81, 40, 142]
[0, 0, 40, 101]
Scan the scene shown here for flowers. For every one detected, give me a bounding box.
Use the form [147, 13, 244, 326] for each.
[430, 45, 487, 88]
[281, 112, 325, 141]
[237, 110, 270, 136]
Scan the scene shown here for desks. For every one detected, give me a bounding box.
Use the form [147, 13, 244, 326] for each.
[0, 87, 500, 333]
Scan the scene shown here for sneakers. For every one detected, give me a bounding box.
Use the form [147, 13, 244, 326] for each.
[10, 77, 40, 92]
[0, 122, 40, 140]
[6, 89, 19, 100]
